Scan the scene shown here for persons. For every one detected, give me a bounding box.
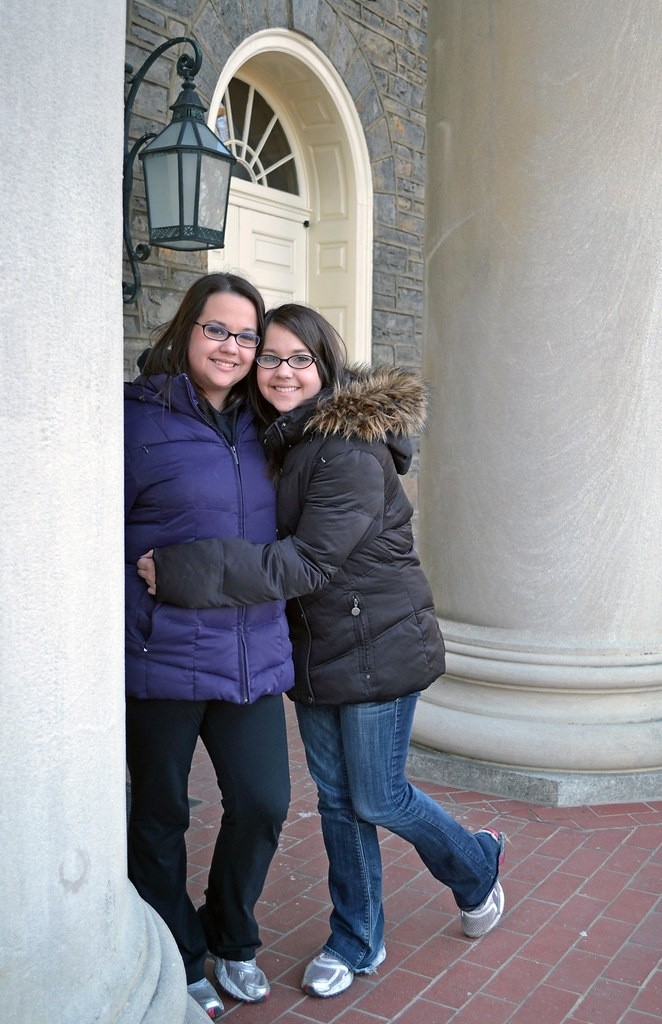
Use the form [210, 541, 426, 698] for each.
[125, 272, 297, 1019]
[136, 303, 510, 998]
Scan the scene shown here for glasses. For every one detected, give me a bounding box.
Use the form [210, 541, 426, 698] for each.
[254, 354, 320, 369]
[195, 321, 263, 348]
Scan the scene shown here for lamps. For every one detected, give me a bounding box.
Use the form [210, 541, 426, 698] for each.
[123, 37, 237, 305]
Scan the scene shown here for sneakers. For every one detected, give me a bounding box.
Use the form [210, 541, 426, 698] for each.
[207, 950, 271, 1004]
[302, 943, 387, 997]
[187, 977, 226, 1019]
[461, 828, 506, 938]
